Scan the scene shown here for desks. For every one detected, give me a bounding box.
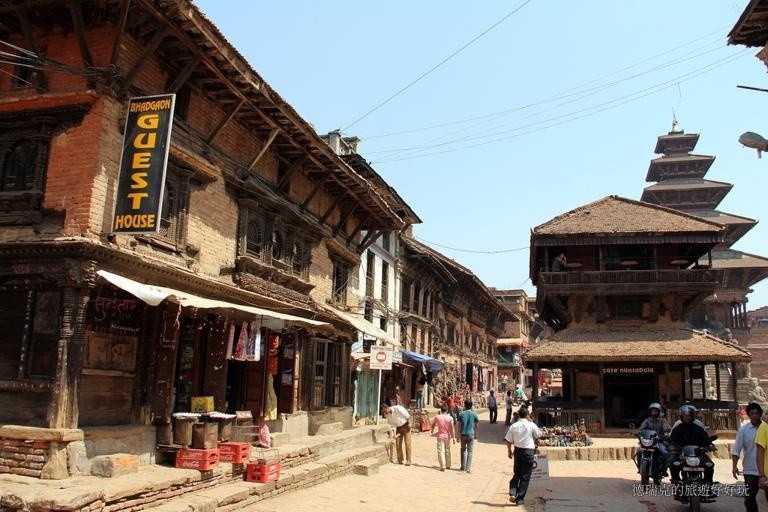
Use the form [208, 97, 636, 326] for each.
[566, 263, 583, 271]
[621, 261, 638, 270]
[670, 260, 689, 269]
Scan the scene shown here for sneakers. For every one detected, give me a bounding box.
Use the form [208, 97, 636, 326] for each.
[662, 470, 668, 477]
[399, 460, 403, 464]
[405, 462, 411, 466]
[509, 495, 516, 503]
[516, 499, 524, 505]
[446, 467, 450, 469]
[440, 468, 444, 472]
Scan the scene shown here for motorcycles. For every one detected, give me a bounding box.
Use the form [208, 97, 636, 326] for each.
[666, 433, 720, 511]
[630, 427, 670, 492]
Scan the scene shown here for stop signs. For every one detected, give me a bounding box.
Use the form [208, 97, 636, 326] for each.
[376, 351, 386, 363]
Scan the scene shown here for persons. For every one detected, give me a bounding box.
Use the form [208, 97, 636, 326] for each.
[512, 386, 522, 406]
[504, 391, 519, 425]
[551, 253, 567, 273]
[504, 406, 542, 505]
[380, 403, 414, 466]
[487, 389, 498, 424]
[456, 398, 479, 474]
[438, 391, 461, 424]
[430, 403, 457, 471]
[668, 403, 719, 499]
[669, 400, 712, 437]
[732, 399, 768, 511]
[501, 372, 508, 391]
[634, 403, 672, 476]
[503, 412, 519, 441]
[755, 411, 768, 503]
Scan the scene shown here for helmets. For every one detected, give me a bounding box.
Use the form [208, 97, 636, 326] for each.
[648, 402, 662, 416]
[678, 404, 697, 419]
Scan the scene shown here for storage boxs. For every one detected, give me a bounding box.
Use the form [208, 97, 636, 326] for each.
[190, 396, 215, 413]
